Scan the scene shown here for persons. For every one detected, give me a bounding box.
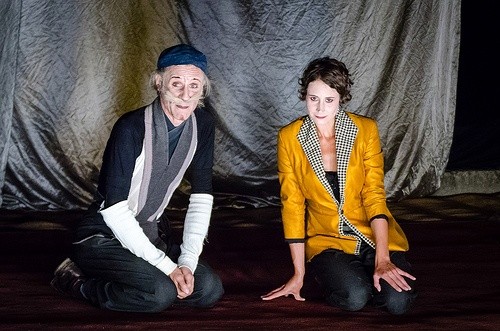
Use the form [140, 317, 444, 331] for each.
[50, 45, 225, 313]
[260, 57, 418, 316]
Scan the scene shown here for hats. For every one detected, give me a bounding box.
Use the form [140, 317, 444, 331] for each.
[157, 44, 207, 74]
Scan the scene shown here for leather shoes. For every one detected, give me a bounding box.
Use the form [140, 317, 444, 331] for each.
[48, 257, 86, 297]
